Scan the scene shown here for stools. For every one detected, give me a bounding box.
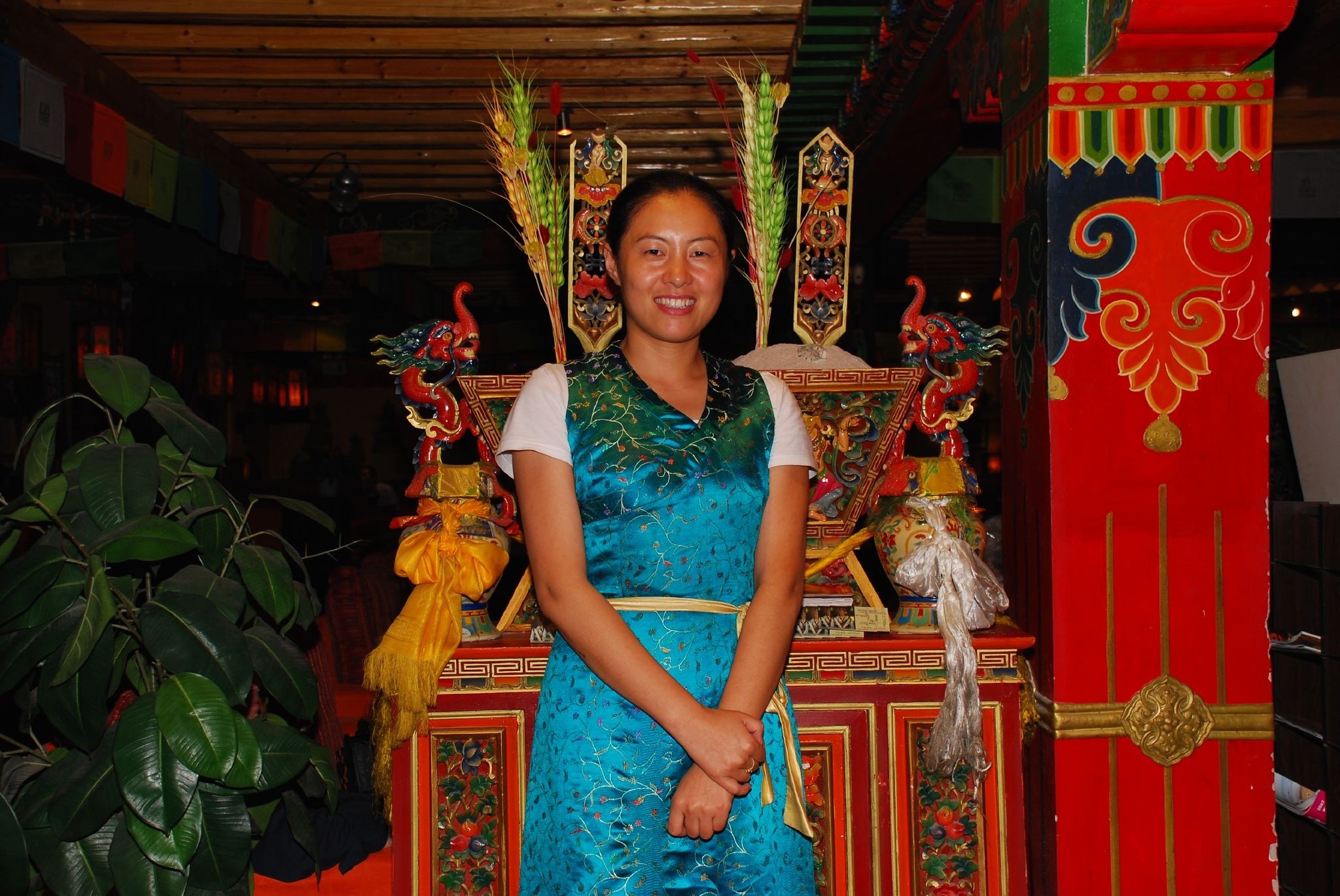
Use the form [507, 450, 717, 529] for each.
[244, 551, 408, 895]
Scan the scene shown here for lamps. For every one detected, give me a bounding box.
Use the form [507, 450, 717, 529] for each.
[292, 152, 359, 212]
[555, 107, 572, 136]
[0, 287, 312, 412]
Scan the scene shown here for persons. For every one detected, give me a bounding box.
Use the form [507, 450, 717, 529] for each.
[493, 169, 818, 896]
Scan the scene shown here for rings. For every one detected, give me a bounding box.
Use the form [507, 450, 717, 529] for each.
[745, 757, 754, 773]
